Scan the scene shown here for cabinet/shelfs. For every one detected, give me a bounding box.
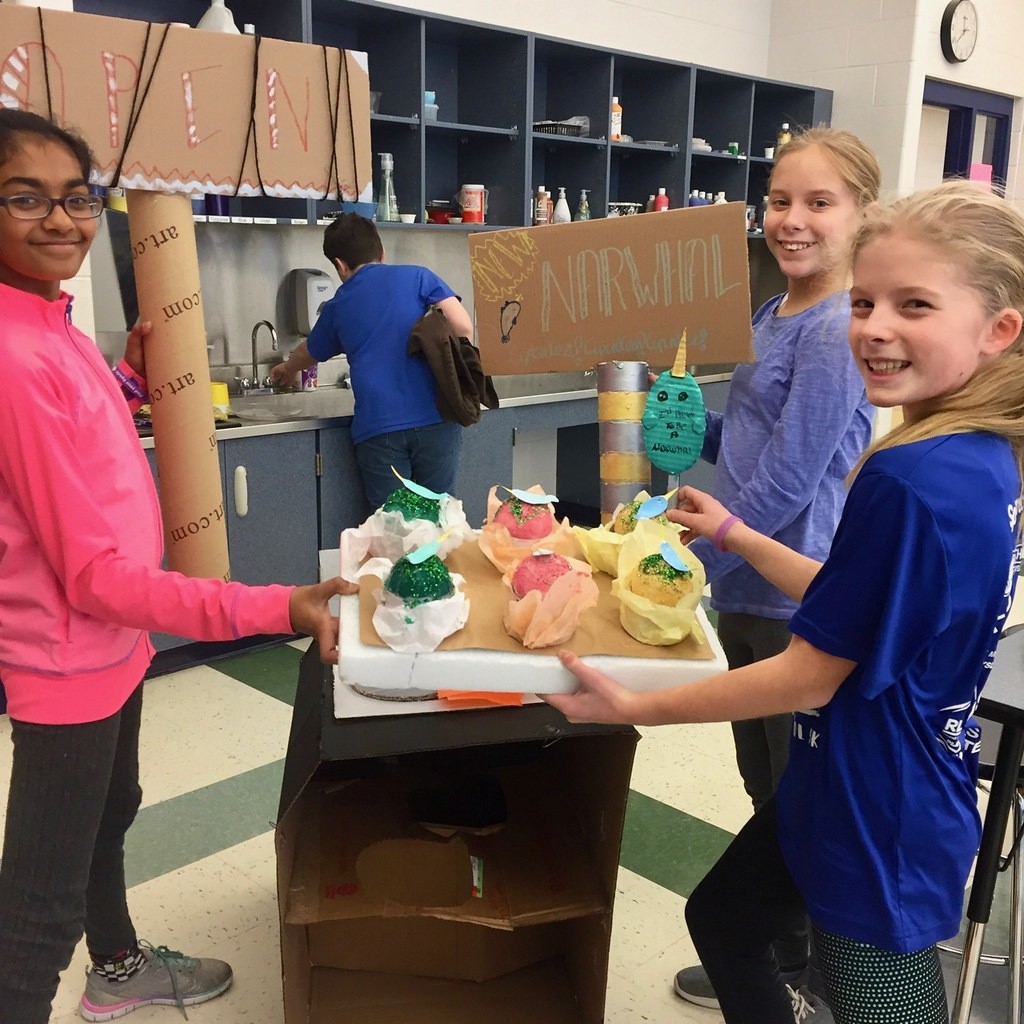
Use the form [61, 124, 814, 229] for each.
[67, 0, 837, 238]
[138, 408, 515, 655]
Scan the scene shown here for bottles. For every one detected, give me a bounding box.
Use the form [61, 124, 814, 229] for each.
[746, 205, 756, 230]
[195, 0, 255, 36]
[645, 188, 669, 212]
[777, 123, 791, 151]
[299, 337, 319, 391]
[530, 185, 554, 227]
[758, 195, 769, 234]
[288, 350, 302, 391]
[610, 96, 622, 141]
[389, 196, 399, 222]
[688, 190, 725, 207]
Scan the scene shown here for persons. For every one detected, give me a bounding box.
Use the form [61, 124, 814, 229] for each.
[537, 178, 1024, 1024]
[674, 128, 881, 1009]
[0, 101, 360, 1024]
[270, 211, 474, 518]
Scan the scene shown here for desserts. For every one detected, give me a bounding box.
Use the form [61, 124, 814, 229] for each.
[385, 463, 696, 611]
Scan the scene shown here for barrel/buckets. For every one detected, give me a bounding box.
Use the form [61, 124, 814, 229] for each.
[460, 184, 489, 224]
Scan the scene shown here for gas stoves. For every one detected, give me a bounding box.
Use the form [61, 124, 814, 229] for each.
[131, 408, 242, 438]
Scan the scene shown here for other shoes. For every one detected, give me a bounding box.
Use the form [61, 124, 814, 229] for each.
[674, 964, 721, 1010]
[785, 982, 836, 1024]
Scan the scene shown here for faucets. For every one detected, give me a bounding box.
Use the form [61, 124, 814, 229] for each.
[250, 319, 279, 389]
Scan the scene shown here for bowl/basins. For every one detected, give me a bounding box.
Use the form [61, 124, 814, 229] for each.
[447, 217, 463, 224]
[425, 91, 436, 104]
[425, 104, 439, 121]
[399, 214, 416, 224]
[370, 90, 383, 114]
[340, 201, 379, 221]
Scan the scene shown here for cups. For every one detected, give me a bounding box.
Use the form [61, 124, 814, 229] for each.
[764, 148, 774, 159]
[210, 381, 231, 416]
[425, 210, 428, 224]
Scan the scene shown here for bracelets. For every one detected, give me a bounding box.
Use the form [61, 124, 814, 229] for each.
[715, 515, 744, 551]
[111, 366, 147, 401]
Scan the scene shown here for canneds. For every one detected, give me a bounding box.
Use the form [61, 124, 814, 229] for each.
[462, 184, 485, 225]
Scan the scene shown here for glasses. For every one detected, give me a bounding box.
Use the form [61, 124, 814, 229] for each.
[0, 194, 107, 220]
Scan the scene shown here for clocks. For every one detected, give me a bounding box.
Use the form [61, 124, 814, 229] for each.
[940, 0, 979, 64]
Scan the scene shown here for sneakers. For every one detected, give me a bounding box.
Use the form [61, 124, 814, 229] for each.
[78, 938, 233, 1021]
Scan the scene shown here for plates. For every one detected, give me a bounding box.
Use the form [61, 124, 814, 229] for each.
[637, 140, 669, 147]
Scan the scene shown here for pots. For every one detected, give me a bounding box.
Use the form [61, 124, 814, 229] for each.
[426, 200, 459, 224]
